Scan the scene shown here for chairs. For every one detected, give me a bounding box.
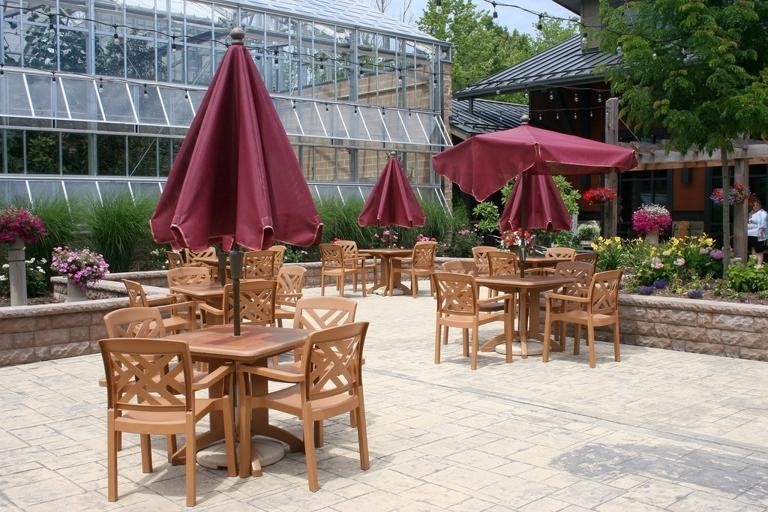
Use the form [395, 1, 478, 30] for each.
[487, 251, 518, 319]
[431, 272, 513, 370]
[99, 307, 208, 465]
[244, 250, 277, 280]
[390, 241, 437, 298]
[545, 247, 576, 259]
[267, 295, 357, 447]
[543, 269, 624, 368]
[319, 243, 367, 297]
[269, 245, 286, 275]
[121, 278, 196, 334]
[574, 252, 598, 276]
[539, 261, 595, 352]
[334, 240, 377, 292]
[441, 260, 504, 357]
[238, 321, 370, 492]
[166, 265, 211, 316]
[472, 246, 499, 277]
[97, 337, 237, 507]
[186, 247, 217, 264]
[197, 279, 279, 328]
[167, 250, 185, 270]
[275, 265, 307, 329]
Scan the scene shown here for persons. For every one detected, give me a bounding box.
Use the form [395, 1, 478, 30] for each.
[747, 199, 767, 267]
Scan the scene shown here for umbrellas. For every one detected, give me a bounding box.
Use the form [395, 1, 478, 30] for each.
[433, 115, 639, 203]
[357, 150, 426, 228]
[149, 27, 325, 251]
[499, 172, 571, 232]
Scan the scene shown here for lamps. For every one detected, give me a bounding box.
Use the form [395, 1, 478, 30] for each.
[185, 94, 189, 104]
[549, 91, 554, 100]
[539, 114, 542, 121]
[274, 58, 280, 70]
[590, 111, 593, 118]
[51, 76, 57, 85]
[493, 12, 499, 25]
[496, 86, 501, 95]
[556, 113, 559, 120]
[319, 64, 325, 76]
[143, 90, 148, 99]
[48, 23, 56, 38]
[574, 113, 578, 119]
[0, 69, 4, 78]
[99, 84, 104, 93]
[616, 42, 623, 53]
[574, 93, 579, 103]
[359, 70, 365, 80]
[114, 33, 120, 46]
[536, 24, 543, 36]
[581, 32, 589, 44]
[598, 93, 602, 103]
[606, 111, 610, 117]
[524, 90, 529, 99]
[398, 75, 403, 85]
[433, 79, 439, 89]
[464, 84, 470, 93]
[172, 44, 176, 54]
[436, 0, 442, 14]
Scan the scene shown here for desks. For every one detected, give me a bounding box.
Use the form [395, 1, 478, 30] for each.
[358, 249, 413, 297]
[160, 324, 324, 479]
[516, 257, 572, 276]
[193, 256, 230, 283]
[170, 283, 224, 326]
[474, 274, 579, 359]
